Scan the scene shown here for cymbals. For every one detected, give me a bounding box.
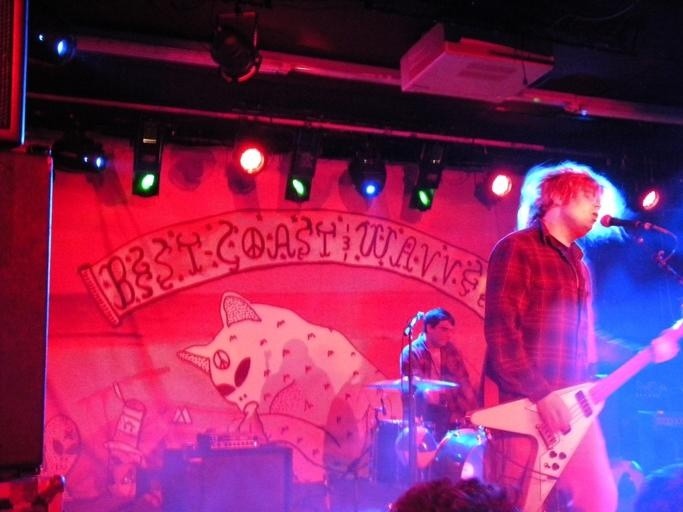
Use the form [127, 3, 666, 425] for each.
[368, 375, 460, 392]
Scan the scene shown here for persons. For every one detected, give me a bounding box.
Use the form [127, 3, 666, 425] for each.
[399, 306, 479, 438]
[482, 159, 622, 512]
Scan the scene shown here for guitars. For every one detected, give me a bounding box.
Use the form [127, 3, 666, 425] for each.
[465, 318, 681, 512]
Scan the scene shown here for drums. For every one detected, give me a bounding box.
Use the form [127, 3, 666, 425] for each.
[372, 420, 422, 488]
[428, 428, 486, 480]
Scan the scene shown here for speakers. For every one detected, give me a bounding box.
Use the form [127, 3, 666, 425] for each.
[379, 418, 410, 478]
[0, 149, 54, 483]
[162, 446, 294, 512]
[0, 0, 28, 149]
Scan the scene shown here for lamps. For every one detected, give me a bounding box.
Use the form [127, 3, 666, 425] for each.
[204, 23, 263, 86]
[28, 99, 662, 211]
[37, 31, 77, 65]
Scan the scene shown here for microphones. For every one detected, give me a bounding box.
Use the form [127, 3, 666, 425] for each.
[380, 398, 386, 416]
[601, 215, 654, 231]
[404, 311, 424, 336]
